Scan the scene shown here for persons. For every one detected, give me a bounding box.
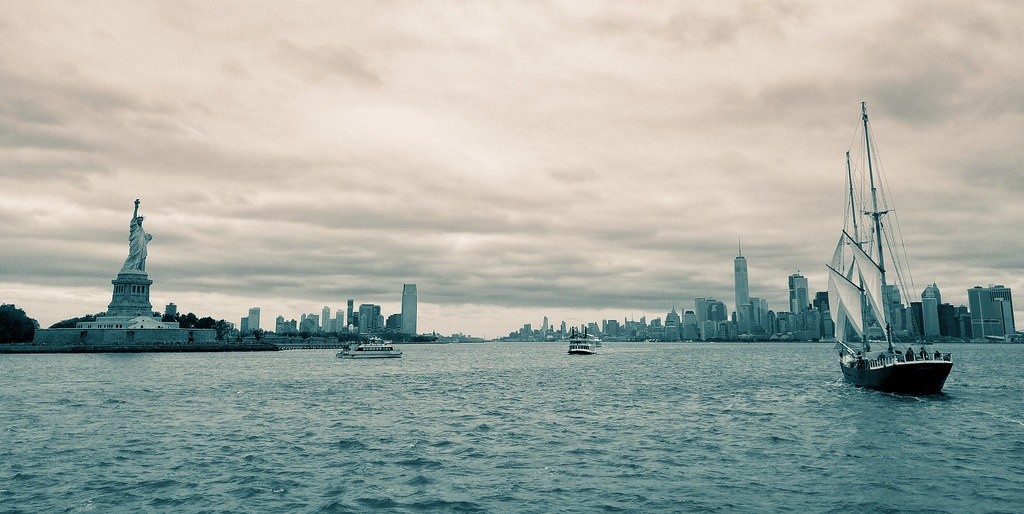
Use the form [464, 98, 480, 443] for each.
[112, 340, 256, 347]
[117, 198, 155, 280]
[894, 347, 952, 364]
[854, 355, 865, 371]
[838, 348, 845, 362]
[877, 350, 886, 366]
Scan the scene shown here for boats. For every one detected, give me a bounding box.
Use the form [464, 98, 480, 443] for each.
[595, 337, 602, 347]
[568, 326, 597, 354]
[336, 337, 402, 359]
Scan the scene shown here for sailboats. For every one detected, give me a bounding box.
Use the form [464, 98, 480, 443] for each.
[824, 101, 954, 395]
